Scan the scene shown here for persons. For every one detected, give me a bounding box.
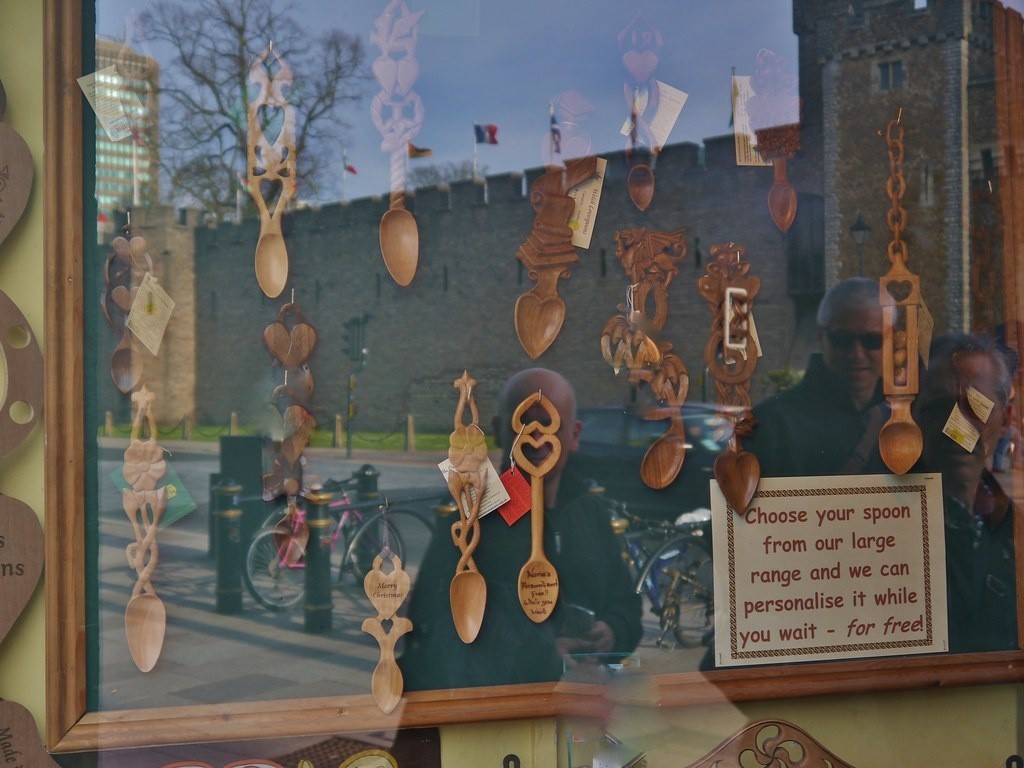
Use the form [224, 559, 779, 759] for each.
[702, 277, 927, 562]
[388, 367, 644, 699]
[698, 333, 1017, 670]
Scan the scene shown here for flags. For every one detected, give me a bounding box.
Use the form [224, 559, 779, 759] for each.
[551, 114, 562, 153]
[408, 144, 431, 158]
[237, 177, 253, 193]
[343, 156, 358, 175]
[473, 123, 497, 144]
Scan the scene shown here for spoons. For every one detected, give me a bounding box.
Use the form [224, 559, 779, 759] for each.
[120, 382, 167, 674]
[361, 547, 414, 716]
[447, 369, 488, 644]
[98, 6, 806, 517]
[512, 393, 559, 623]
[879, 122, 924, 474]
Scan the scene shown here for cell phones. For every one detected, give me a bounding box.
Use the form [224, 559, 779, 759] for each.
[561, 603, 596, 661]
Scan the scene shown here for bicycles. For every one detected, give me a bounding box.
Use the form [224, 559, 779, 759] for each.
[587, 480, 714, 652]
[243, 468, 405, 612]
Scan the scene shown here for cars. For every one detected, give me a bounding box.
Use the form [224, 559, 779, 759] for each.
[565, 403, 752, 504]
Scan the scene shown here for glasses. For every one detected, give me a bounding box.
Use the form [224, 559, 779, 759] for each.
[821, 326, 883, 351]
